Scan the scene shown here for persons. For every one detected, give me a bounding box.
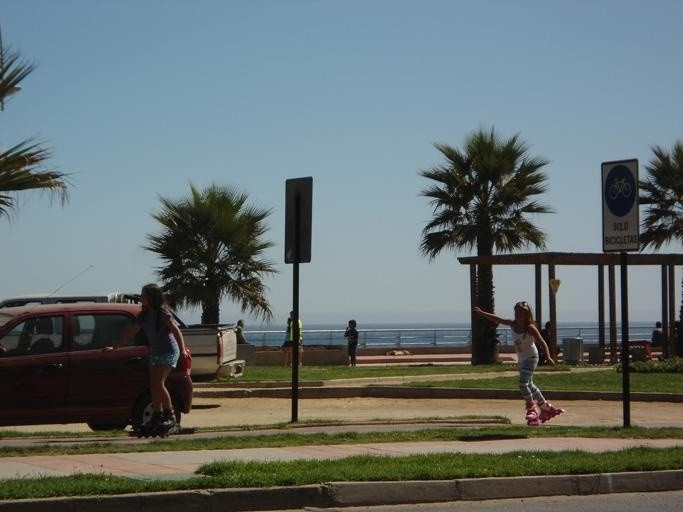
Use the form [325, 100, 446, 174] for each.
[103, 284, 189, 432]
[235, 319, 249, 345]
[540, 321, 552, 347]
[473, 301, 556, 421]
[281, 317, 291, 366]
[344, 320, 359, 366]
[652, 322, 662, 347]
[289, 311, 303, 368]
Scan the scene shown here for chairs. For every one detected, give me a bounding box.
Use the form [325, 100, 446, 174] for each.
[30, 316, 83, 353]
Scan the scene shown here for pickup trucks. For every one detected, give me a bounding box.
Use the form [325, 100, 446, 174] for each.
[1, 288, 246, 382]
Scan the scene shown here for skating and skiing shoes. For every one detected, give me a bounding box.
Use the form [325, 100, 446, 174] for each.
[525, 405, 539, 425]
[153, 412, 177, 438]
[135, 413, 163, 438]
[538, 401, 565, 423]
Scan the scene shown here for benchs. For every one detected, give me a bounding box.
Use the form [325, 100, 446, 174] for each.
[556, 342, 663, 362]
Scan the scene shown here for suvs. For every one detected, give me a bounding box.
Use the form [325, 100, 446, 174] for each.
[0, 303, 194, 437]
